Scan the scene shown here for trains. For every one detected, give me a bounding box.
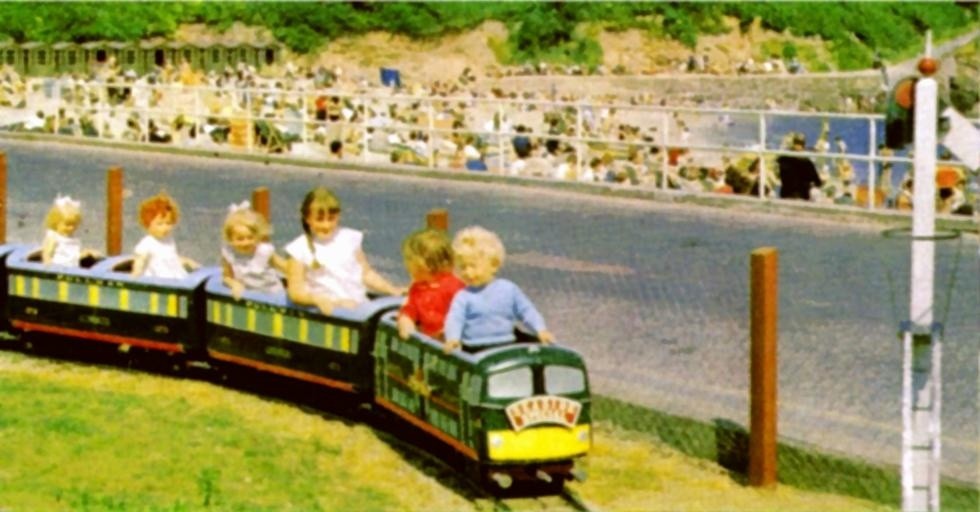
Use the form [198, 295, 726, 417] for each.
[0, 238, 597, 499]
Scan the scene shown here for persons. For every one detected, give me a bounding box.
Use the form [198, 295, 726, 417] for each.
[898, 150, 975, 215]
[439, 224, 560, 357]
[219, 202, 289, 303]
[124, 191, 193, 281]
[885, 56, 941, 150]
[280, 185, 410, 320]
[38, 186, 90, 270]
[394, 224, 470, 340]
[0, 40, 895, 216]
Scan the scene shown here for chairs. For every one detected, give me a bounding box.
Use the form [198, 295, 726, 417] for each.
[23, 61, 973, 215]
